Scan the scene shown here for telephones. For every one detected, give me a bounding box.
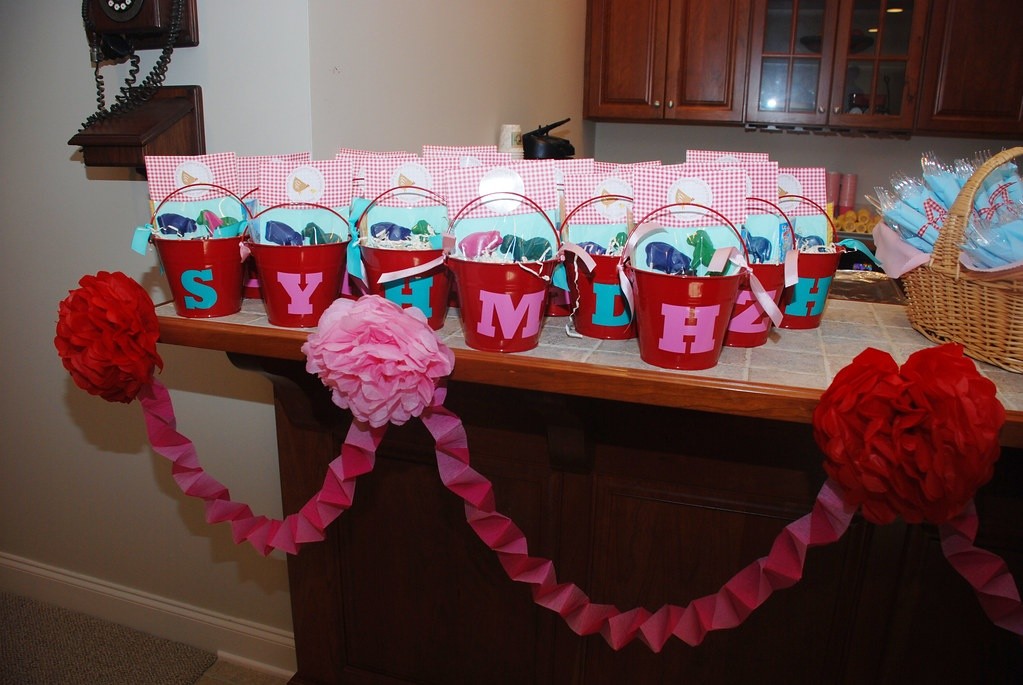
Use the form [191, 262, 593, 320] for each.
[77, 1, 200, 61]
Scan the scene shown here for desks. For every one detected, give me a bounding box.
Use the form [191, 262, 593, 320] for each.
[145, 271, 1022, 685]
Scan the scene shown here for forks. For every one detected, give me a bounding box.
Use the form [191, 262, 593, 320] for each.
[863, 145, 1023, 268]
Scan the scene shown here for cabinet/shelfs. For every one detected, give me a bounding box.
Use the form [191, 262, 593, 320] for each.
[909, 0, 1022, 144]
[582, 0, 752, 128]
[744, 1, 927, 136]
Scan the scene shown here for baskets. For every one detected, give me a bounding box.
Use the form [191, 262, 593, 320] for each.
[875, 136, 1023, 377]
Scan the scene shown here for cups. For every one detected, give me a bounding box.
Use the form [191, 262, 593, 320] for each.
[825, 169, 880, 234]
[498, 124, 525, 160]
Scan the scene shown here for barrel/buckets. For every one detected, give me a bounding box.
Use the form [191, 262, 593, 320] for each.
[774, 193, 843, 330]
[356, 185, 451, 331]
[339, 177, 365, 300]
[238, 187, 262, 299]
[441, 192, 565, 353]
[560, 194, 637, 340]
[450, 279, 460, 308]
[721, 198, 796, 349]
[241, 202, 353, 331]
[618, 203, 751, 371]
[147, 184, 255, 318]
[548, 182, 572, 317]
